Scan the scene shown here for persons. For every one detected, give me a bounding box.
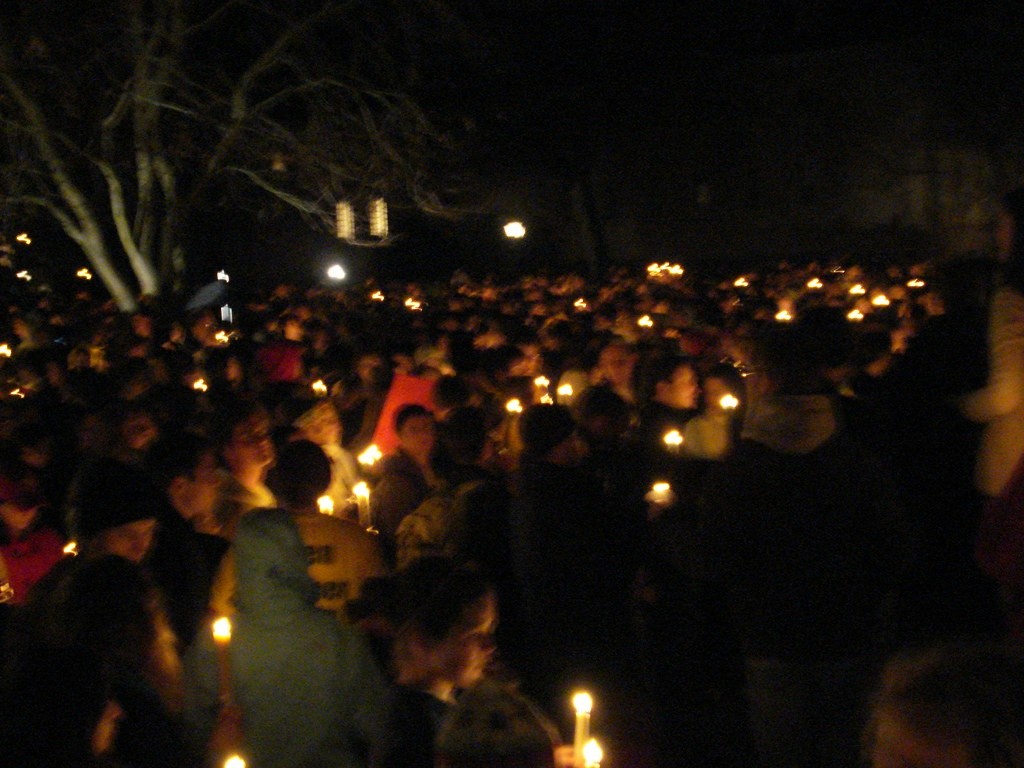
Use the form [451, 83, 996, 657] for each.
[0, 218, 1024, 767]
